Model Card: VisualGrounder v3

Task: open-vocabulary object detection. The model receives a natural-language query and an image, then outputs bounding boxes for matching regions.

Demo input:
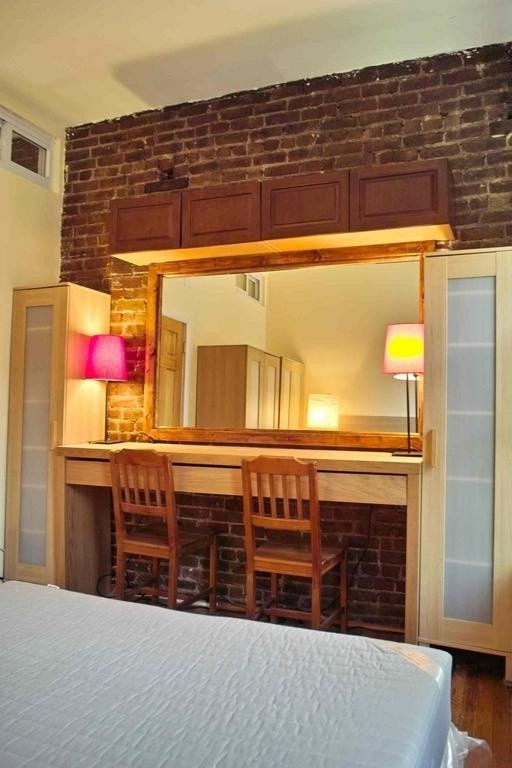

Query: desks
[56,443,422,645]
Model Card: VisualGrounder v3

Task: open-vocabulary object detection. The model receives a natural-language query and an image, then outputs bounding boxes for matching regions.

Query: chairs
[111,448,217,614]
[242,455,347,631]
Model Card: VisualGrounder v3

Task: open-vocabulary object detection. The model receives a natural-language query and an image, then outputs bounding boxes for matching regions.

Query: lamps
[383,324,427,457]
[80,334,123,444]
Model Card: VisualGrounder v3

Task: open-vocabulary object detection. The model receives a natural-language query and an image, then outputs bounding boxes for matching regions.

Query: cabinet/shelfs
[349,157,456,241]
[260,172,350,241]
[108,193,181,256]
[181,181,260,251]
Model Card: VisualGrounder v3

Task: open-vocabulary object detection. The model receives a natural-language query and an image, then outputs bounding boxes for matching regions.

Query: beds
[0,580,457,767]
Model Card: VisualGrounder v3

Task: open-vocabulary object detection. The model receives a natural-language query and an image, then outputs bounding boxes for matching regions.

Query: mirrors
[143,237,438,452]
